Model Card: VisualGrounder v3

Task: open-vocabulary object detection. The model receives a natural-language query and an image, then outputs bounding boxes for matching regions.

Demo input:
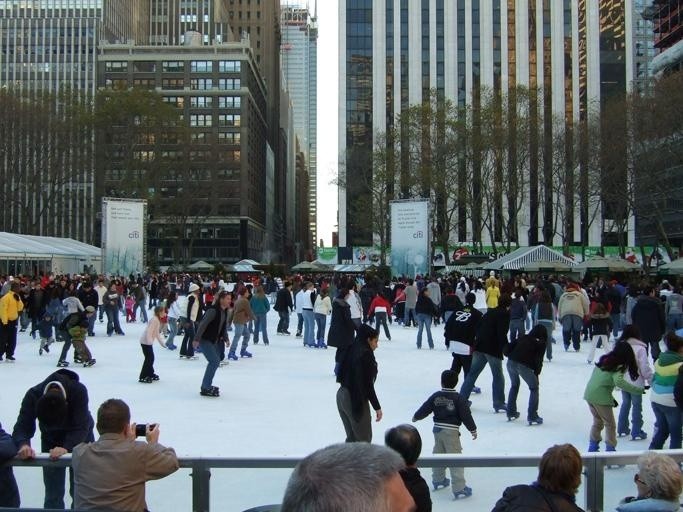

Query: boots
[303,339,325,346]
[228,352,238,359]
[240,349,253,355]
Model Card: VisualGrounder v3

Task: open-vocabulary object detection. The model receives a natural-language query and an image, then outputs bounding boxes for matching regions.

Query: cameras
[135,424,152,436]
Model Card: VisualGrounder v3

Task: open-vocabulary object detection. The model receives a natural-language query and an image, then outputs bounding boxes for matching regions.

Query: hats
[189,282,200,292]
[86,306,95,313]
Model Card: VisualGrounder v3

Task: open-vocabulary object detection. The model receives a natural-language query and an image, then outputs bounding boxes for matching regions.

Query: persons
[0,269,683,512]
[625,247,637,264]
[358,250,366,260]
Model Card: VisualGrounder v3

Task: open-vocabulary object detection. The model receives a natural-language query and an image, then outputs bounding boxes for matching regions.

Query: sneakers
[139,374,159,380]
[632,430,646,437]
[495,404,508,410]
[433,478,449,486]
[507,412,519,418]
[618,428,630,433]
[473,387,480,392]
[529,416,542,421]
[45,344,49,352]
[277,328,282,332]
[296,330,301,336]
[455,487,471,494]
[58,361,68,365]
[6,355,15,360]
[166,344,176,349]
[283,330,290,334]
[202,386,220,395]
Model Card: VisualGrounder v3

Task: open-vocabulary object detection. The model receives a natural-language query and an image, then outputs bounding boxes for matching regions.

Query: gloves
[14,294,20,301]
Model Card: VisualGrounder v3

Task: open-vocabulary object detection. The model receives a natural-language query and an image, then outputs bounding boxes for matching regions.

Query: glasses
[634,473,646,485]
